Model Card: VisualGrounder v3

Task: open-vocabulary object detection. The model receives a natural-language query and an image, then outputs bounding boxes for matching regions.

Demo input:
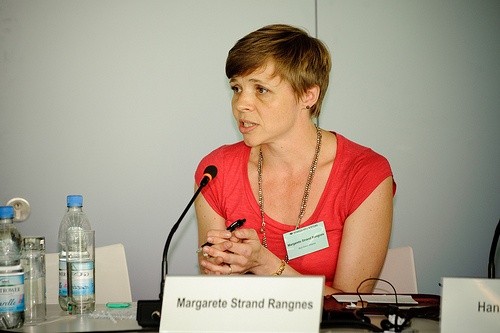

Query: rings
[229,266,232,273]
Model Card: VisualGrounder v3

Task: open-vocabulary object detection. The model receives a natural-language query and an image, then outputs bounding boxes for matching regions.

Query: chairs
[374,243,419,296]
[43,245,132,305]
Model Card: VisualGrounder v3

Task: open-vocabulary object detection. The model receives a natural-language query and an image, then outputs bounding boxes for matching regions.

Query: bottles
[0,205,25,330]
[58,195,92,311]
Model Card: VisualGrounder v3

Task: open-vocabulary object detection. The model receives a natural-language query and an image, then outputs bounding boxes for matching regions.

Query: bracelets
[273,260,286,276]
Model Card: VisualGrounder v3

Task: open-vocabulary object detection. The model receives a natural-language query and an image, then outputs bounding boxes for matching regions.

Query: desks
[0,301,440,333]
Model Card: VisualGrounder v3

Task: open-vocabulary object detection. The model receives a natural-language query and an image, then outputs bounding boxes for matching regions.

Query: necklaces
[257,128,321,263]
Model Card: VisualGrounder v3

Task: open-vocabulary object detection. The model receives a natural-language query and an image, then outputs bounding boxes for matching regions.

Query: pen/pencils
[196,218,246,253]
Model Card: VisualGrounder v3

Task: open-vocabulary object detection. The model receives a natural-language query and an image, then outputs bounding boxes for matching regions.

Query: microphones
[135,165,218,329]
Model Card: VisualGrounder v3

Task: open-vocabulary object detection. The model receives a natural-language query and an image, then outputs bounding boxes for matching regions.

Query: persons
[195,24,397,297]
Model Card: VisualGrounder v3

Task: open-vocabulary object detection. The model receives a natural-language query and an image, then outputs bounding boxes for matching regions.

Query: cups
[21,236,46,326]
[66,231,96,315]
